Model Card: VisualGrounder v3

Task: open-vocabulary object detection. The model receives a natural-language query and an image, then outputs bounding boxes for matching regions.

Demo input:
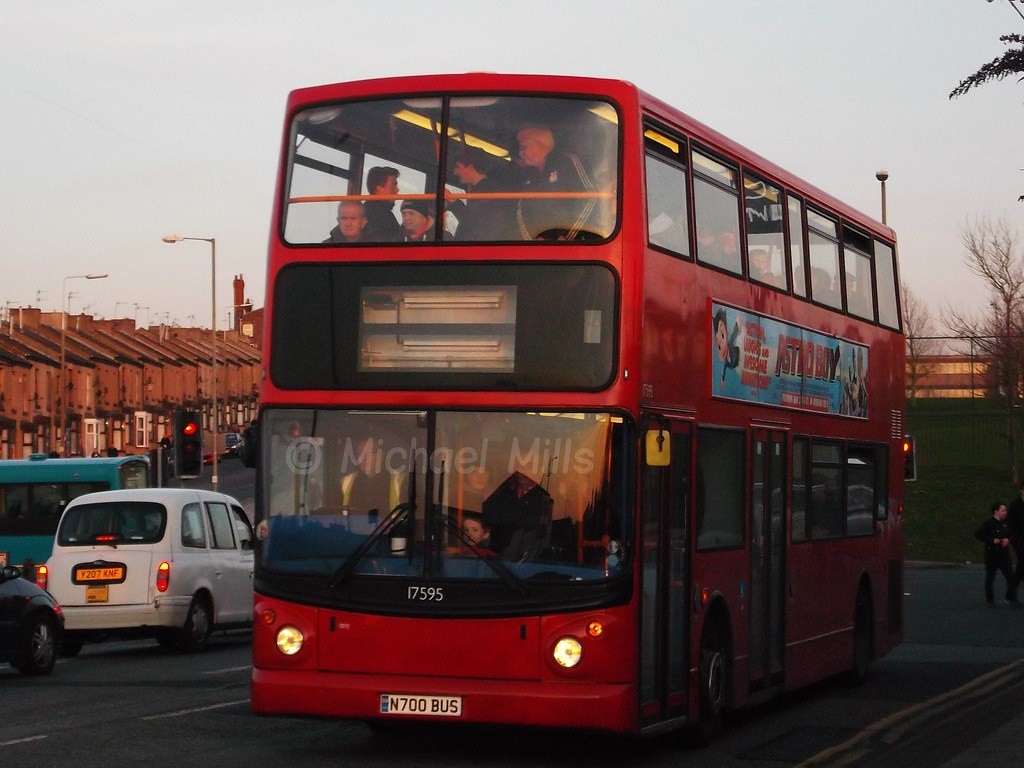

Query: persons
[451,457,496,517]
[444,517,496,557]
[750,249,783,288]
[515,127,601,241]
[482,453,553,561]
[334,436,408,517]
[321,201,382,243]
[363,167,400,242]
[1004,488,1024,602]
[388,199,454,241]
[974,502,1014,607]
[443,147,515,240]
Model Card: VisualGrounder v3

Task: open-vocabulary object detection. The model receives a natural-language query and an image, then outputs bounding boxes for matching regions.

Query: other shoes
[986,600,996,608]
[1004,600,1022,608]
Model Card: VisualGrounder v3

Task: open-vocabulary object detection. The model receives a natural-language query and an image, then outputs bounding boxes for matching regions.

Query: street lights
[163,236,218,491]
[223,304,253,432]
[59,273,108,459]
[875,172,889,226]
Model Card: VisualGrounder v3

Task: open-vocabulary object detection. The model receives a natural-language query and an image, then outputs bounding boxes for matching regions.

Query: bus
[0,453,150,578]
[253,72,907,741]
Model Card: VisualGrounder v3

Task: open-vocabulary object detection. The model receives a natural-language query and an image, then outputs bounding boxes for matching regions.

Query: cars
[202,451,222,465]
[221,433,245,459]
[0,564,65,678]
[36,487,255,654]
[846,485,886,535]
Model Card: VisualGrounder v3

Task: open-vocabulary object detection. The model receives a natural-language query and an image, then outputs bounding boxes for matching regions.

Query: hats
[403,199,428,216]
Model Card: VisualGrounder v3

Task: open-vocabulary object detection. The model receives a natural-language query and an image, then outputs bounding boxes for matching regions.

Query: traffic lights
[175,412,203,478]
[905,435,917,482]
